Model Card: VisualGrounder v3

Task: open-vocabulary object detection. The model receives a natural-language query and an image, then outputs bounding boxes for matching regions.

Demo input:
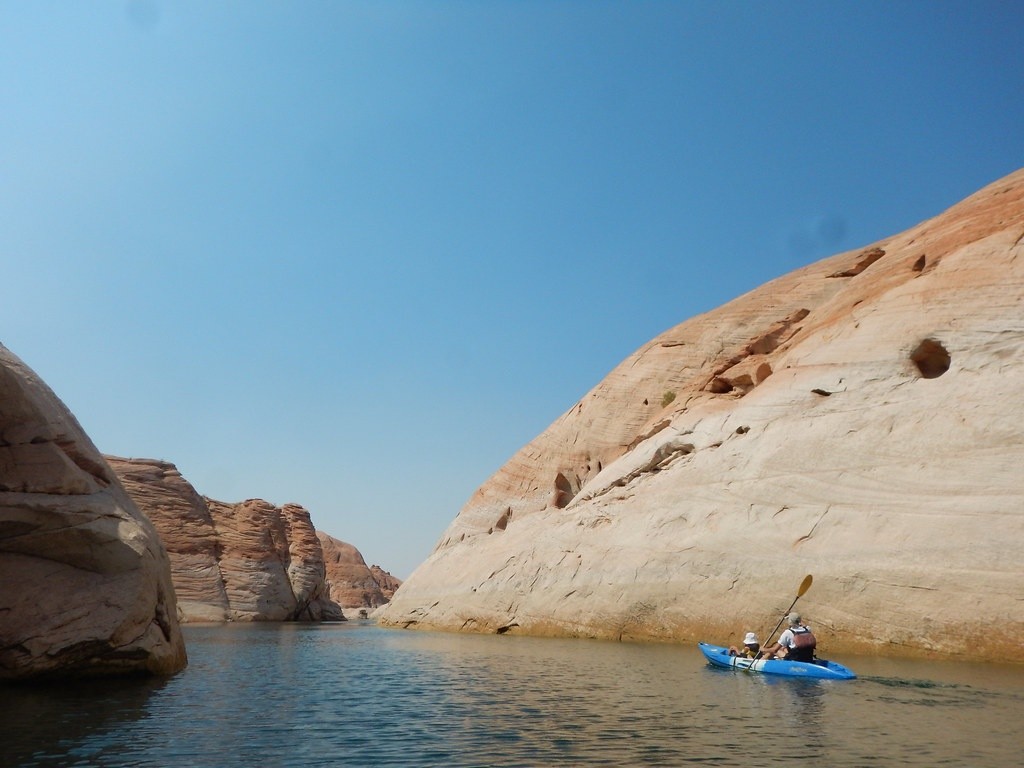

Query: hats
[742,632,758,644]
[785,612,802,624]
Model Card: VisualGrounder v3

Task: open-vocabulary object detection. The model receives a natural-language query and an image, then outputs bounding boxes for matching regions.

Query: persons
[759,612,816,663]
[729,633,763,659]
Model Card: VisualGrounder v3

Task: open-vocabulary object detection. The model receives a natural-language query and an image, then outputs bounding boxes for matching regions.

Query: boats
[696,641,856,679]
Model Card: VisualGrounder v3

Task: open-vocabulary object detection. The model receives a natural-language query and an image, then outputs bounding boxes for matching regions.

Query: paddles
[743,573,813,673]
